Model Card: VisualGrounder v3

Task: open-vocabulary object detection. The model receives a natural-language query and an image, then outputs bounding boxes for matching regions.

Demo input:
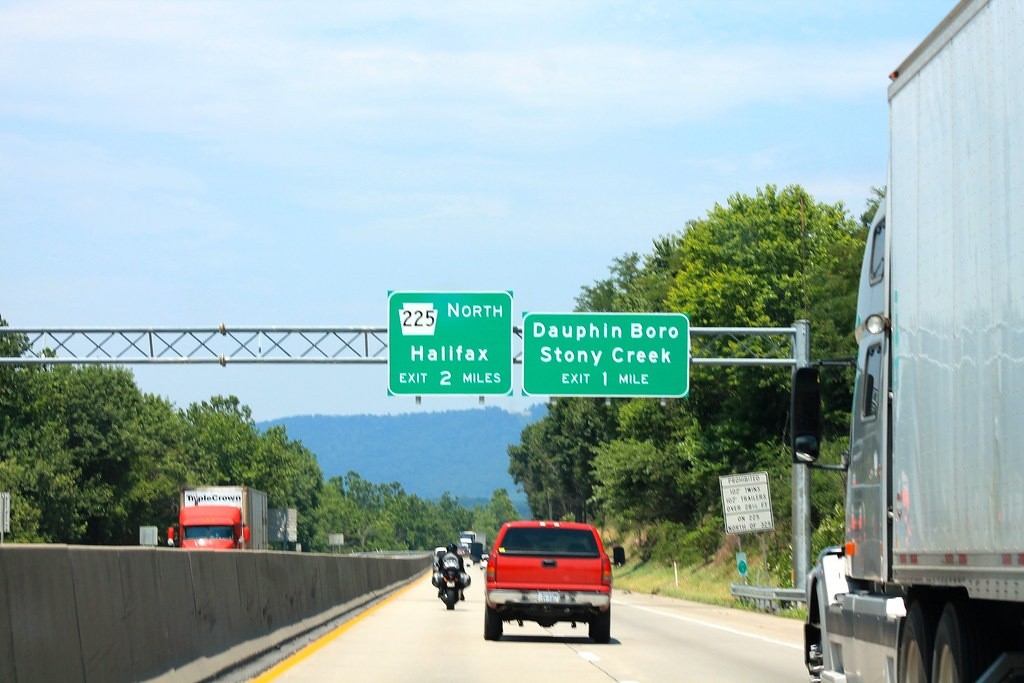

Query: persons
[437,544,466,600]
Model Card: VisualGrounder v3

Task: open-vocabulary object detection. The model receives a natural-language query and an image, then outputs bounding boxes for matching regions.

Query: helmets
[446,543,457,552]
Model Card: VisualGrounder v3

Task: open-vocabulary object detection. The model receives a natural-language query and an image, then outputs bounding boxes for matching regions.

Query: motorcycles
[433,553,461,610]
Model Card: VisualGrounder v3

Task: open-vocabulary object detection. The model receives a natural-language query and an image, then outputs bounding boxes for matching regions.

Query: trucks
[790,0,1022,683]
[167,485,268,551]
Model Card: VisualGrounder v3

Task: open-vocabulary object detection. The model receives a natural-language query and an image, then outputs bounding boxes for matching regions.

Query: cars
[469,519,625,641]
